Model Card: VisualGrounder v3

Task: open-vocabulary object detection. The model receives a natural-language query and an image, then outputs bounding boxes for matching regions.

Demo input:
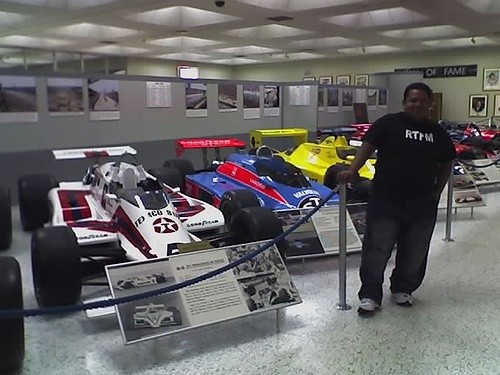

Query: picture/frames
[493,94,500,117]
[354,74,369,86]
[469,94,488,117]
[319,76,333,85]
[483,68,500,91]
[336,75,351,85]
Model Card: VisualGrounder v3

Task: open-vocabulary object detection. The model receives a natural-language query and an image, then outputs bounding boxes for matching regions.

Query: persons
[227,241,296,312]
[487,72,498,86]
[336,82,458,313]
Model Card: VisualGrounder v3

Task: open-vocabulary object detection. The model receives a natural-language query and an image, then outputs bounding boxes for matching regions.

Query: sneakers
[390,291,414,306]
[357,297,383,315]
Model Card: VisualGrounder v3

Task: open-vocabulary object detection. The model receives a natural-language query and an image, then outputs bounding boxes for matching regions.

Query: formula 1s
[249,127,379,199]
[18,145,286,308]
[350,119,500,171]
[175,140,372,263]
[125,302,183,331]
[114,272,167,290]
